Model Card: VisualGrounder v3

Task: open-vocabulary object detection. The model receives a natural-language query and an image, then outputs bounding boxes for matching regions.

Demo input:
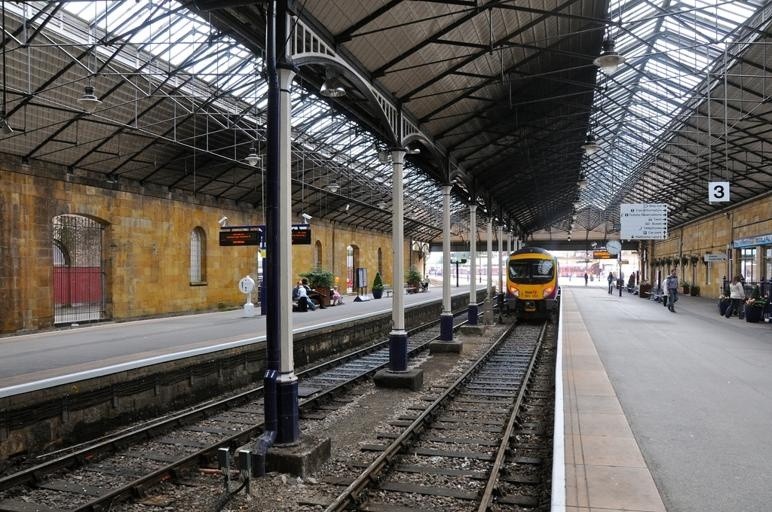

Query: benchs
[385,289,393,298]
[330,295,344,304]
[405,287,416,295]
[615,280,668,308]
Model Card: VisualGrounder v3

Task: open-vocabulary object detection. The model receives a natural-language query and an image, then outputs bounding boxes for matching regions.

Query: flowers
[716,294,730,303]
[741,282,765,309]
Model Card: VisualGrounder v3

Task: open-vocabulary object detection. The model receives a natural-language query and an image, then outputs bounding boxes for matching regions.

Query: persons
[423,274,431,284]
[607,271,613,295]
[584,272,588,286]
[290,278,344,310]
[723,274,745,319]
[666,268,679,311]
[661,272,670,307]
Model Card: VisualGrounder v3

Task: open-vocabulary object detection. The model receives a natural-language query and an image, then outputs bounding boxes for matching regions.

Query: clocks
[606,240,622,256]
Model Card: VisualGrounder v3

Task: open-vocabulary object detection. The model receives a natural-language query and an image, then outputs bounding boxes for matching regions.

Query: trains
[505,246,559,322]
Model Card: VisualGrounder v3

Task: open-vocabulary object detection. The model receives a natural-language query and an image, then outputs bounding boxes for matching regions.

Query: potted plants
[404,264,422,294]
[689,284,700,297]
[677,278,689,294]
[298,265,336,309]
[372,270,384,299]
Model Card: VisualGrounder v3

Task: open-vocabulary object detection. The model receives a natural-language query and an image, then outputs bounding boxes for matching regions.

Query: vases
[719,301,728,316]
[745,304,763,323]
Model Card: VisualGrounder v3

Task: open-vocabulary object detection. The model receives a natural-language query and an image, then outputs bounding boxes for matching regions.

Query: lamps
[327,178,341,194]
[244,145,262,169]
[318,68,347,100]
[579,126,604,160]
[589,30,630,75]
[75,83,105,117]
[564,172,592,243]
[376,197,388,210]
